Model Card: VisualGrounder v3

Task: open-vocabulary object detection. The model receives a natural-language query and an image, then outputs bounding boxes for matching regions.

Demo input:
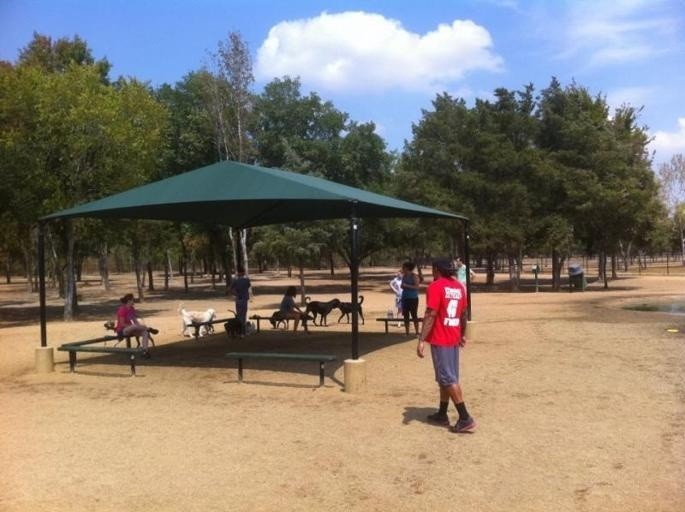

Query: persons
[389,266,407,328]
[454,255,476,289]
[416,254,478,433]
[279,284,309,337]
[225,264,257,339]
[400,261,421,338]
[112,292,158,362]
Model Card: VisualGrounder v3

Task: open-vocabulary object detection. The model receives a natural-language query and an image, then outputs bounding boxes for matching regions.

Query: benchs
[376,318,424,332]
[188,317,235,341]
[58,346,146,376]
[64,334,132,349]
[225,353,335,385]
[249,317,309,332]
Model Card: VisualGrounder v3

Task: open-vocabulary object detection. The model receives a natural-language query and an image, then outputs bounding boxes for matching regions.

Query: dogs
[177,301,216,339]
[104,319,159,350]
[333,296,364,325]
[301,296,341,326]
[269,311,286,329]
[224,309,242,338]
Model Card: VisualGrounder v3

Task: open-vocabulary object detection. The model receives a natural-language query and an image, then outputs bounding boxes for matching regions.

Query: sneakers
[428,414,449,426]
[451,418,474,432]
[149,328,158,334]
